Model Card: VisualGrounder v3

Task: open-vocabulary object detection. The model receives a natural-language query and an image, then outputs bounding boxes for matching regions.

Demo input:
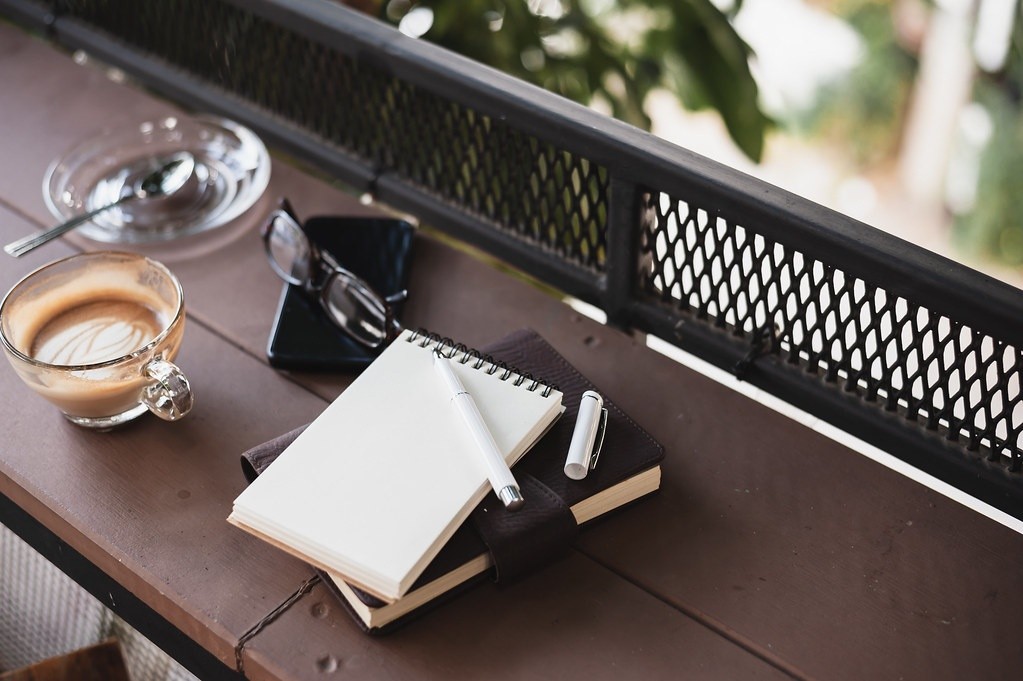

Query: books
[222,322,668,634]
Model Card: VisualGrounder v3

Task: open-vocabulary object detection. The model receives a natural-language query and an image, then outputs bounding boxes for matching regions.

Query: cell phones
[265,213,411,373]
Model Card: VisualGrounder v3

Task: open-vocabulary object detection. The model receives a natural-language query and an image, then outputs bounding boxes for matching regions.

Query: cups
[0,250,194,433]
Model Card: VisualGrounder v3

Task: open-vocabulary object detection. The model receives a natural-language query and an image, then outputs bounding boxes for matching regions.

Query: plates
[42,112,272,247]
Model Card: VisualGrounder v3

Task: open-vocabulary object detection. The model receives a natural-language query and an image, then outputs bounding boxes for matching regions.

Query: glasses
[263,197,408,348]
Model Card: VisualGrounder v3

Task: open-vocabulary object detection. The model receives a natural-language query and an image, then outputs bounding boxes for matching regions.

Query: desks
[0,25,1023,681]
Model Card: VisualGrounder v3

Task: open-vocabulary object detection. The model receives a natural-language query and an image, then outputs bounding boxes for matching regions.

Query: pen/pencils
[430,346,524,510]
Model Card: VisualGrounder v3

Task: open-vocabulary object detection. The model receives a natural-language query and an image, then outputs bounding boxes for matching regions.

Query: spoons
[3,151,195,258]
